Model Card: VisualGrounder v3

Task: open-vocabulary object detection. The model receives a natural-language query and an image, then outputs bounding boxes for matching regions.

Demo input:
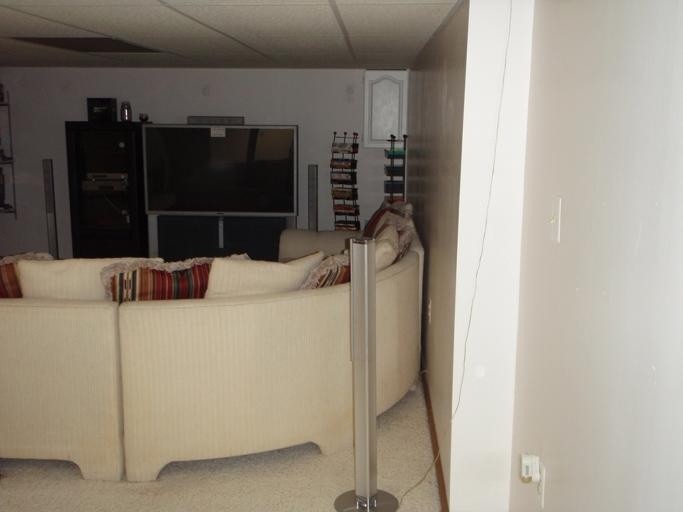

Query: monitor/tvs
[138,122,299,217]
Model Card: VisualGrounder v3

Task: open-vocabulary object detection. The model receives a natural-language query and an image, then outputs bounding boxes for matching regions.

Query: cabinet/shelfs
[62,113,148,257]
[0,90,23,221]
[329,128,361,233]
[383,132,411,202]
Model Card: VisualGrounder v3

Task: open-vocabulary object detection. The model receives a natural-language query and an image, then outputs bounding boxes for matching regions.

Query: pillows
[97,257,207,302]
[360,200,416,268]
[307,252,350,289]
[14,254,162,300]
[0,252,45,300]
[206,252,321,296]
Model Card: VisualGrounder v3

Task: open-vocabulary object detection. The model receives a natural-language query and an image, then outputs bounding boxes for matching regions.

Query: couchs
[0,228,424,483]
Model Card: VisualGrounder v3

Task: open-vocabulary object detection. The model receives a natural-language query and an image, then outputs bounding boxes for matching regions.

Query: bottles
[120,101,132,122]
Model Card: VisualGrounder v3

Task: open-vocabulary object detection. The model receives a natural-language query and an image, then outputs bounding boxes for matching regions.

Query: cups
[138,112,148,121]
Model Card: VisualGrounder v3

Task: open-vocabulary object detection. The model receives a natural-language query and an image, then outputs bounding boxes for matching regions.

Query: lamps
[40,157,58,257]
[333,235,399,512]
[307,163,319,234]
